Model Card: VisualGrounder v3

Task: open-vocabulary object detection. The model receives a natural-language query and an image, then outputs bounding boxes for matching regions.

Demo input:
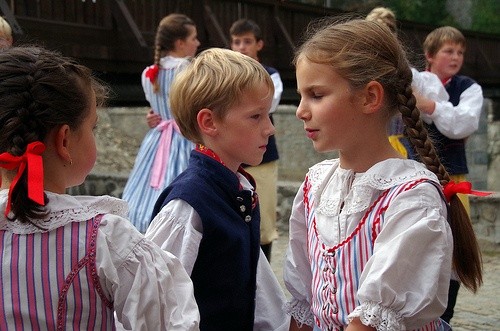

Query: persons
[0,15,13,49]
[280,20,484,331]
[0,45,202,331]
[146,19,283,264]
[366,7,451,126]
[410,27,484,223]
[121,13,200,234]
[144,48,290,331]
